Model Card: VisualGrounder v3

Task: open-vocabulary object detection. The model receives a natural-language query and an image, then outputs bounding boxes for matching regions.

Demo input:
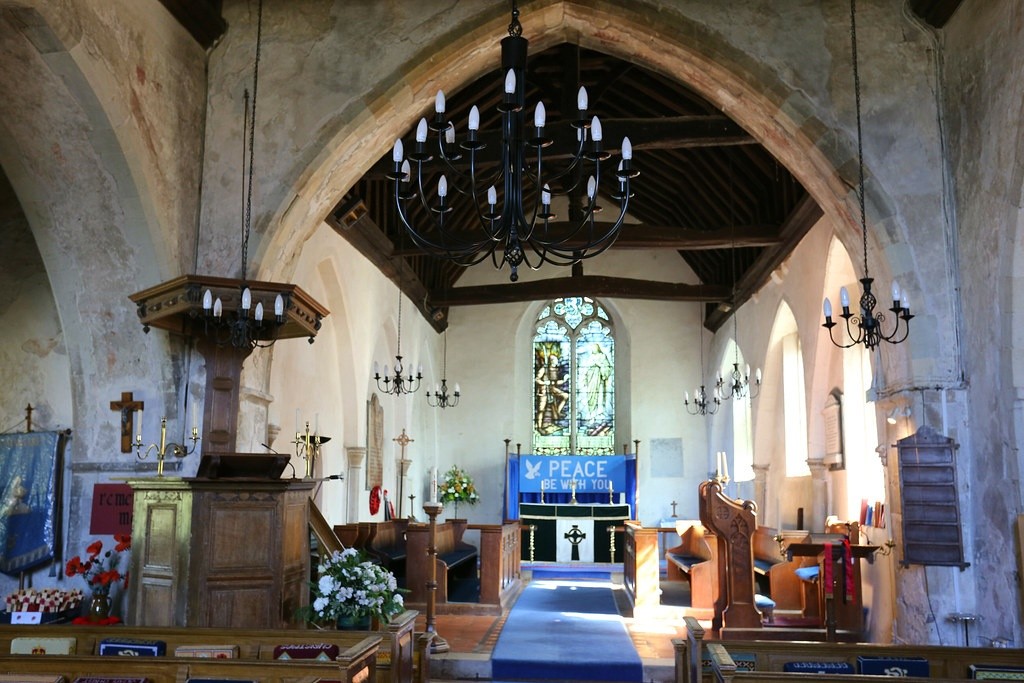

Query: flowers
[66,533,133,594]
[293,544,406,619]
[440,464,484,514]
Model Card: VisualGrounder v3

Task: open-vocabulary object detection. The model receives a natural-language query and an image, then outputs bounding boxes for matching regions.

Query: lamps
[386,0,642,283]
[683,303,721,416]
[199,0,288,350]
[425,330,465,408]
[714,210,762,400]
[821,0,916,350]
[717,302,733,313]
[370,254,426,395]
[431,308,445,322]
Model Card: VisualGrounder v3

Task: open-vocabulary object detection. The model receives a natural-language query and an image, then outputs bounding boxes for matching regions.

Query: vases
[336,607,373,630]
[90,589,119,622]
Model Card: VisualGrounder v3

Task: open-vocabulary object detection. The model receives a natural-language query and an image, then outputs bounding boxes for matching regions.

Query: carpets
[490,567,645,683]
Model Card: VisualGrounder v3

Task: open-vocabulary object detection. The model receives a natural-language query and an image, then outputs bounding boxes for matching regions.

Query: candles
[295,407,302,434]
[716,450,722,477]
[136,406,143,435]
[314,412,320,435]
[541,480,544,489]
[723,451,728,476]
[609,479,613,490]
[193,401,198,426]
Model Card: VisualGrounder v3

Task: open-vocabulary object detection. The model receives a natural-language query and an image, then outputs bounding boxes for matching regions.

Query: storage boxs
[173,644,240,658]
[700,650,759,674]
[98,636,167,657]
[271,642,338,661]
[968,663,1024,680]
[10,636,76,656]
[0,607,82,624]
[782,658,856,674]
[857,655,930,677]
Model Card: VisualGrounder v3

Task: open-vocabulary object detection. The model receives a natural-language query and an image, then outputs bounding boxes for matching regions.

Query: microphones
[262,443,295,477]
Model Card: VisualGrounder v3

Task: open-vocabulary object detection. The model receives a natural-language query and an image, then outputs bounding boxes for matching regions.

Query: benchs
[754,593,777,623]
[667,519,720,608]
[754,525,808,609]
[334,521,406,577]
[409,517,478,604]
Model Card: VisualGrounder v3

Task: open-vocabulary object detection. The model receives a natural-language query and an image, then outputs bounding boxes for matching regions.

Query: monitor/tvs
[197,453,291,479]
